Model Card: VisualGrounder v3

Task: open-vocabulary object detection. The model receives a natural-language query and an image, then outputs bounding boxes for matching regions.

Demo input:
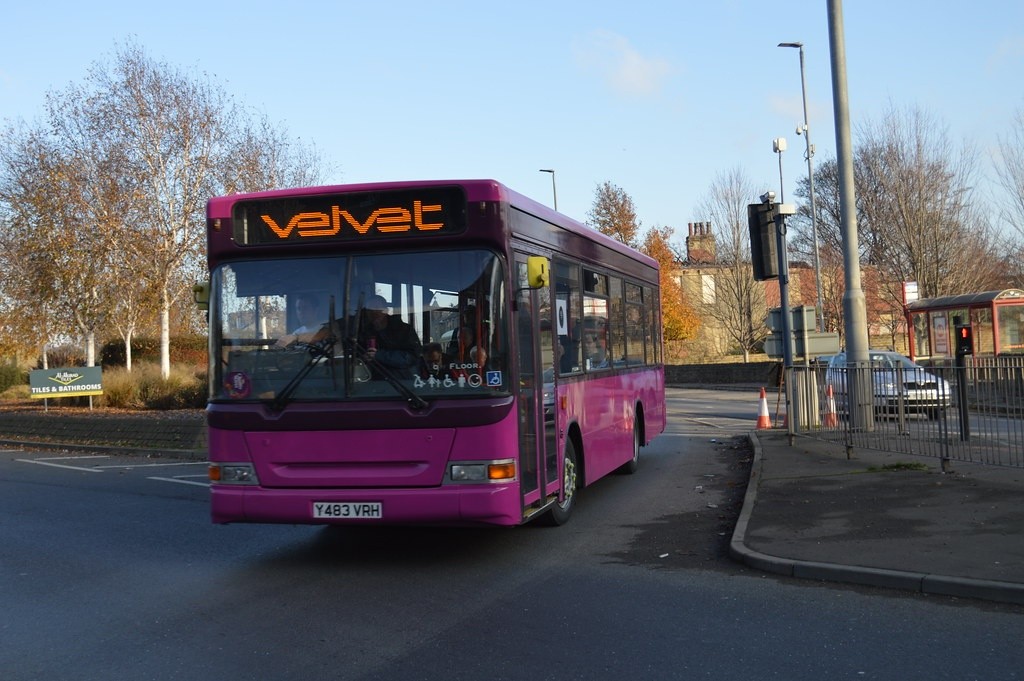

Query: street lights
[539,169,557,211]
[780,43,824,331]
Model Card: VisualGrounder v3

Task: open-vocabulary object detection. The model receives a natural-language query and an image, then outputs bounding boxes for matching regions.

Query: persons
[421,342,469,381]
[627,308,644,339]
[451,328,486,366]
[584,334,608,368]
[276,294,342,357]
[451,306,476,340]
[560,336,578,373]
[366,296,419,380]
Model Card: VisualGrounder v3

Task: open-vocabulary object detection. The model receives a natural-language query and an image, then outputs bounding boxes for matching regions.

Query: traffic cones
[757,387,772,429]
[782,413,789,429]
[823,384,839,426]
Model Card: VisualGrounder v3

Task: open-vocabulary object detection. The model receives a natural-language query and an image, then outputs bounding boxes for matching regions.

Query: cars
[829,351,952,420]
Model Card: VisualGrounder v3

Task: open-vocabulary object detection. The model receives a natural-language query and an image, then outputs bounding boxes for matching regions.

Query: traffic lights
[958,325,974,355]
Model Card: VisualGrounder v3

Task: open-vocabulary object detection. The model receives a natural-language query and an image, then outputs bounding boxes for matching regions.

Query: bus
[192,180,667,528]
